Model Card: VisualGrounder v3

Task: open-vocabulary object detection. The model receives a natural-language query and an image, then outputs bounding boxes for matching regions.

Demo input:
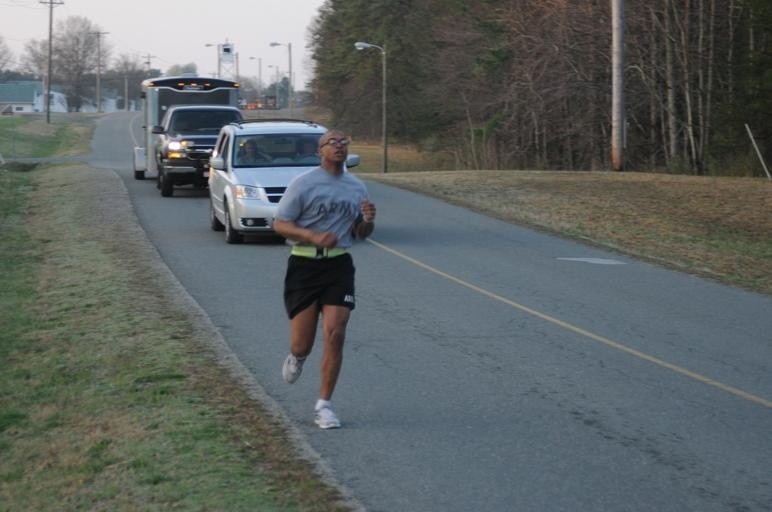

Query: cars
[152,105,245,197]
[208,120,360,244]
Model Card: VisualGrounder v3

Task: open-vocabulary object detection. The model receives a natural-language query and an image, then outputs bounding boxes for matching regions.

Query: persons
[273,129,376,429]
[237,140,270,166]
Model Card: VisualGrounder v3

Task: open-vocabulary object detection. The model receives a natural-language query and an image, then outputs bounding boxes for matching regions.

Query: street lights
[268,64,279,109]
[205,44,221,75]
[270,42,293,120]
[250,56,261,100]
[355,42,389,174]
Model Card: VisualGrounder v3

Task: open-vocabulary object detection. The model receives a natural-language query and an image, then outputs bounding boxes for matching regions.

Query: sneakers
[315,404,340,430]
[282,353,306,384]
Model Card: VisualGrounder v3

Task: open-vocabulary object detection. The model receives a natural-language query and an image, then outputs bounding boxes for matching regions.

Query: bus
[132,77,239,180]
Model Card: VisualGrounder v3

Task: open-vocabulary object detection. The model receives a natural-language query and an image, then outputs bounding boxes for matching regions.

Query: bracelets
[363,216,374,224]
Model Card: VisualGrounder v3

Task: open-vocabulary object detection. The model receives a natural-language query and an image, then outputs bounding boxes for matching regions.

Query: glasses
[320,137,349,148]
